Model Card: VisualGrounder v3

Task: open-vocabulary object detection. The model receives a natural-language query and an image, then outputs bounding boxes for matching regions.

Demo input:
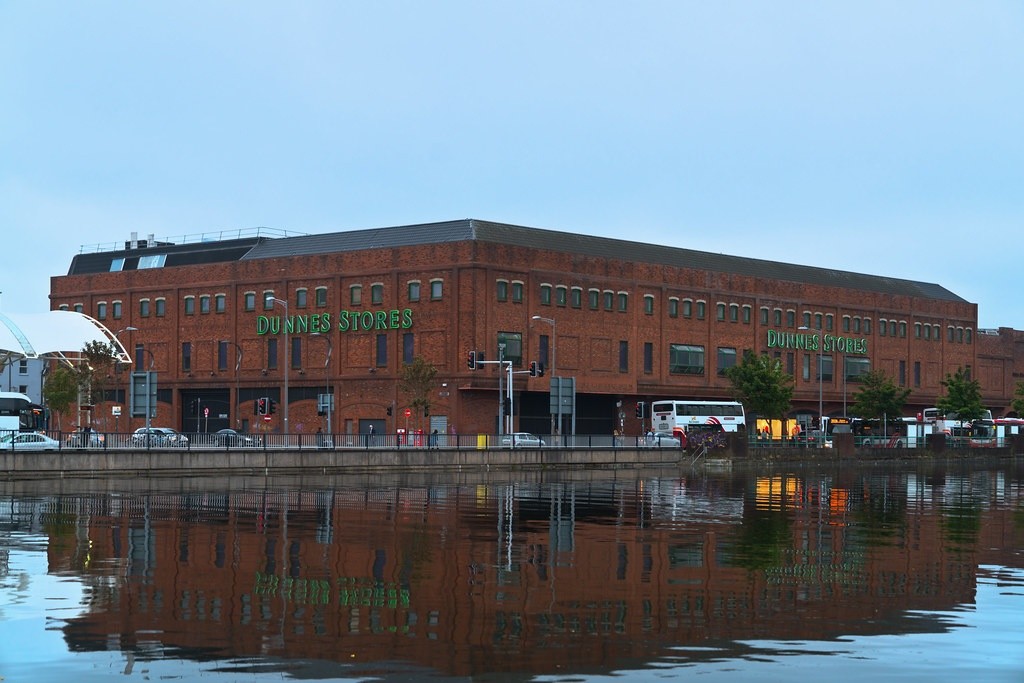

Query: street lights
[532,316,556,447]
[221,341,242,433]
[309,332,332,448]
[265,296,288,447]
[798,326,824,447]
[133,347,154,449]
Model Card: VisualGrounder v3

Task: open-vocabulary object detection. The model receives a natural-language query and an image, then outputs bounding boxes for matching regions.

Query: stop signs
[404,409,410,416]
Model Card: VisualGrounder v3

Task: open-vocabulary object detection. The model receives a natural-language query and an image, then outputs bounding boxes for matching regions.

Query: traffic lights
[503,398,511,415]
[635,402,643,419]
[529,360,536,376]
[259,397,276,416]
[387,405,391,416]
[424,408,428,417]
[468,351,475,369]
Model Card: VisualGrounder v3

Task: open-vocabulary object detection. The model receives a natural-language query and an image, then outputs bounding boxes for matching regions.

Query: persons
[369,425,375,438]
[80,423,94,448]
[296,422,304,432]
[431,430,440,449]
[645,427,656,446]
[316,427,328,447]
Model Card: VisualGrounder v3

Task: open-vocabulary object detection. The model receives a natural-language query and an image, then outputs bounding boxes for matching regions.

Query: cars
[68,429,105,447]
[0,433,59,450]
[210,429,261,448]
[131,427,189,447]
[502,432,545,447]
[797,430,833,448]
[636,432,681,446]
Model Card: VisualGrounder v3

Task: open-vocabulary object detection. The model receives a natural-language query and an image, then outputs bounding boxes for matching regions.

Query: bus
[651,399,746,436]
[817,408,1024,448]
[0,392,46,443]
